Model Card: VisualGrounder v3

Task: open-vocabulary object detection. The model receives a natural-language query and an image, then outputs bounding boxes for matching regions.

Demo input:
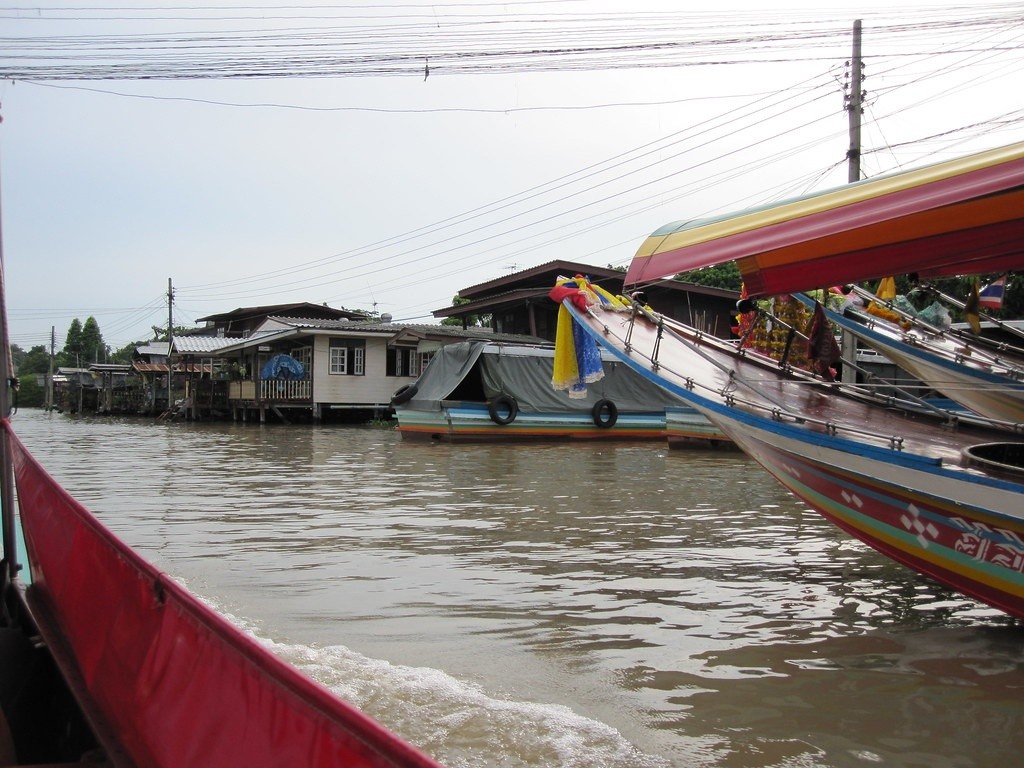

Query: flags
[978,273,1007,308]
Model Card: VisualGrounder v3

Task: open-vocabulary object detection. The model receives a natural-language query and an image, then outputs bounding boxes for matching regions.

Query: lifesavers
[592,399,618,428]
[489,394,518,424]
[391,383,418,405]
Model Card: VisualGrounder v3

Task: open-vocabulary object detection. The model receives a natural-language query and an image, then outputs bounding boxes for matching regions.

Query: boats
[391,336,978,453]
[549,140,1023,626]
